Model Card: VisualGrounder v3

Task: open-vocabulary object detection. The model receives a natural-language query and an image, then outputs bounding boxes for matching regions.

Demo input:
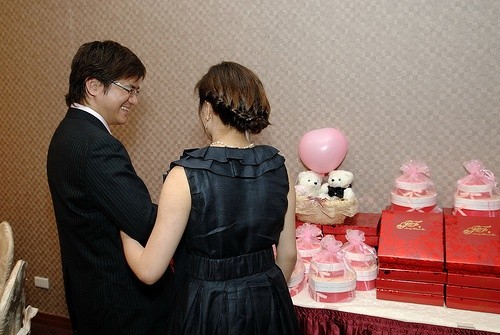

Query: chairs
[0,219,39,335]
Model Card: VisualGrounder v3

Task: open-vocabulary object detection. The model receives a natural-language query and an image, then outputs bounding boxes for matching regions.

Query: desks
[289,281,500,335]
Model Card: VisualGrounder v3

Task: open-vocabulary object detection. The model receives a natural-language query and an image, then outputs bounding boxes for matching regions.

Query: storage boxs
[294,204,500,314]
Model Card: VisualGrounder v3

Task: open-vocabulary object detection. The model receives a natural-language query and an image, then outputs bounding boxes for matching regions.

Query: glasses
[110,79,139,97]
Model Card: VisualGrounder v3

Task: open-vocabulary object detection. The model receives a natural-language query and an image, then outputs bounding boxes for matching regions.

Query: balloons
[298,127,348,174]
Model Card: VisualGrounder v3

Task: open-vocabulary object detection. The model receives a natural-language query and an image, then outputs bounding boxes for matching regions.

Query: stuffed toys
[322,170,355,200]
[295,171,324,198]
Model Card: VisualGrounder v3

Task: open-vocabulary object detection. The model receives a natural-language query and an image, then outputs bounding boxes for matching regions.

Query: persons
[119,62,302,335]
[46,40,174,335]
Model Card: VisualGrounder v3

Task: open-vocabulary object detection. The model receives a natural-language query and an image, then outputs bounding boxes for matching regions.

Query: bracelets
[210,142,254,148]
